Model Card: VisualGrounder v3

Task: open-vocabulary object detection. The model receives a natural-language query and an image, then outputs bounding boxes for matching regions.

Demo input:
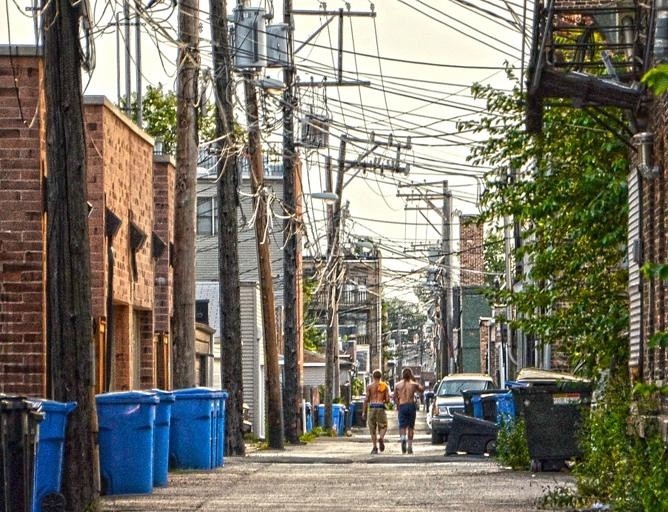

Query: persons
[360,370,391,455]
[393,369,425,454]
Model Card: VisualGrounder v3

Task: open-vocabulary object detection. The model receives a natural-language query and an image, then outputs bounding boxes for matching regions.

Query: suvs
[430,368,497,445]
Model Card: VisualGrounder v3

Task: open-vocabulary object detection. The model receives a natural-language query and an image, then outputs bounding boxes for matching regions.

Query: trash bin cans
[445,381,593,473]
[23,397,78,512]
[95,386,229,495]
[303,402,367,434]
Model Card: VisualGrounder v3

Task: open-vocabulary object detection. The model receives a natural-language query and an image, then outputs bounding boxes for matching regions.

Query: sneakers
[401,440,413,454]
[371,439,384,454]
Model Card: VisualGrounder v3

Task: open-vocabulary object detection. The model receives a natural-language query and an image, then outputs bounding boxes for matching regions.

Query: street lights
[299,192,338,200]
